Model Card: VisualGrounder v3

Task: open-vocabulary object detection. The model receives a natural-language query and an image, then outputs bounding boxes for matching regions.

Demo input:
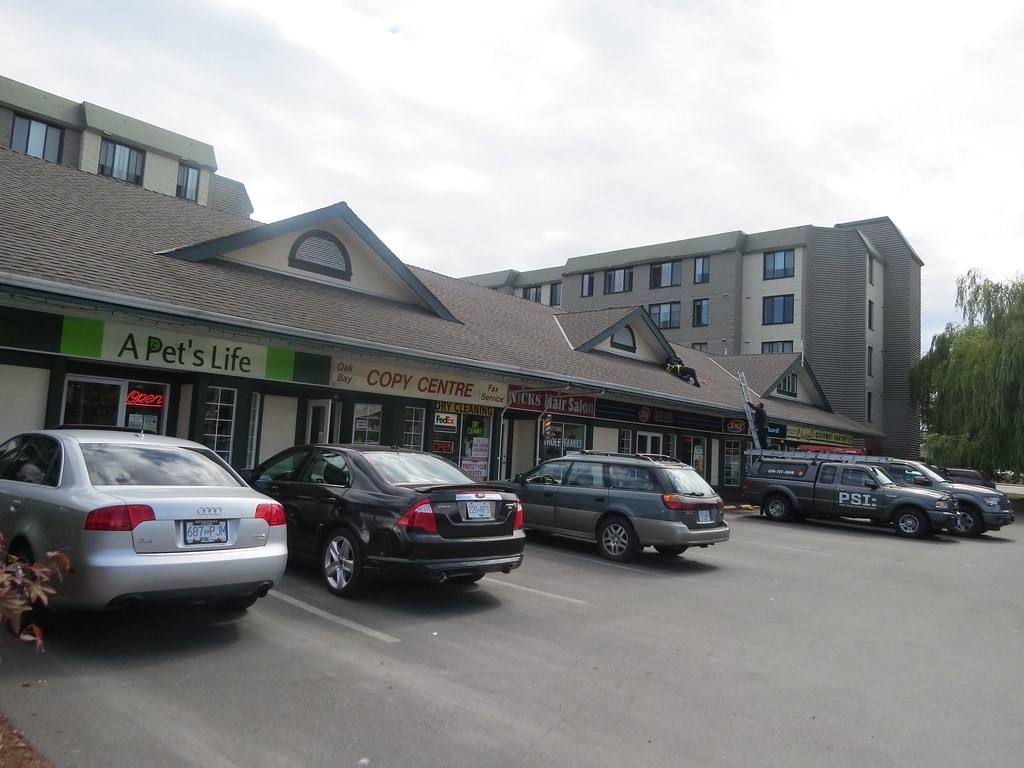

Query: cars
[0,423,288,627]
[243,443,527,598]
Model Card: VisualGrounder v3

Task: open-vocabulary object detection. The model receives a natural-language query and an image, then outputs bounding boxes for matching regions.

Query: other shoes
[681,376,688,382]
[694,382,701,387]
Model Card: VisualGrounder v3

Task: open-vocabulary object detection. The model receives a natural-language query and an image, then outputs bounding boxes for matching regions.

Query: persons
[665,355,701,387]
[747,400,768,448]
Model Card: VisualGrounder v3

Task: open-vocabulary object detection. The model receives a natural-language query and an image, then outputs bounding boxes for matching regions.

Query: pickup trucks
[743,457,959,539]
[857,457,1015,538]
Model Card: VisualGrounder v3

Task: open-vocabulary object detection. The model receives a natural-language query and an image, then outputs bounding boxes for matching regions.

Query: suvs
[484,450,730,562]
[941,467,995,487]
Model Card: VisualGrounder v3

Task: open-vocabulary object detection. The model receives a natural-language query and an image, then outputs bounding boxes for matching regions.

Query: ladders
[737,371,761,449]
